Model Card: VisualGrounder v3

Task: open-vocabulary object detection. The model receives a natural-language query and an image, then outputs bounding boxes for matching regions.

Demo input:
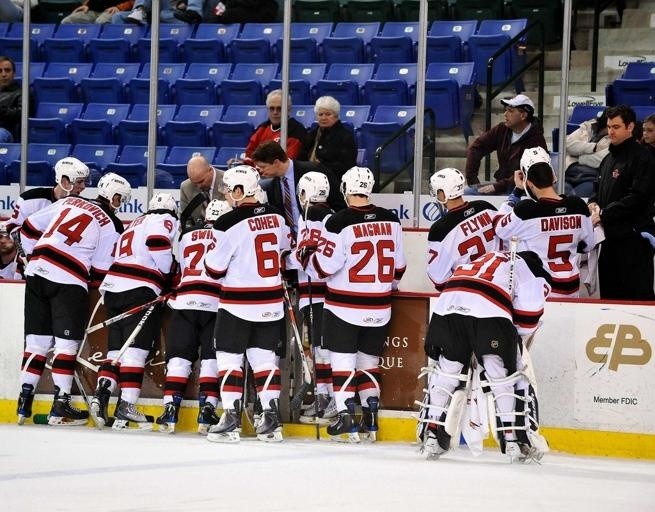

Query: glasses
[270,106,281,113]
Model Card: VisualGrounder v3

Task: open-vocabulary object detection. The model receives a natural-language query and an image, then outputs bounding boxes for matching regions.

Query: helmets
[97,172,132,209]
[340,166,376,198]
[296,171,331,209]
[429,168,465,204]
[0,215,18,239]
[55,157,90,193]
[520,146,557,184]
[205,165,268,222]
[148,192,179,214]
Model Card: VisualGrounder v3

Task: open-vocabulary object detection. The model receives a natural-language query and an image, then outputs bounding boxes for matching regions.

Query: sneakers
[424,436,445,455]
[506,439,531,459]
[127,10,148,24]
[173,9,203,23]
[17,391,34,418]
[298,394,378,435]
[197,402,283,433]
[50,387,182,424]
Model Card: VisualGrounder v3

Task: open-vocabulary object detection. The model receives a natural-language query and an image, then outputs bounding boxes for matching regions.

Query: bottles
[32,413,49,424]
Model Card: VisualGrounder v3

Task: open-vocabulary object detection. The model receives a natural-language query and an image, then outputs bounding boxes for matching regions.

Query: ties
[282,177,294,237]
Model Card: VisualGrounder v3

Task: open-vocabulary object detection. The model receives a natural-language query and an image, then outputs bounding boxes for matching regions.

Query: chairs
[552,60,655,154]
[1,0,549,201]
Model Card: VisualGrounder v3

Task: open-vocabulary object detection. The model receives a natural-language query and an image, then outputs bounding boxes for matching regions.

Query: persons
[0,143,338,444]
[466,93,551,197]
[561,110,614,197]
[284,168,406,443]
[643,113,655,147]
[228,89,307,190]
[1,1,238,24]
[0,55,37,144]
[589,103,654,300]
[294,96,358,213]
[414,146,596,458]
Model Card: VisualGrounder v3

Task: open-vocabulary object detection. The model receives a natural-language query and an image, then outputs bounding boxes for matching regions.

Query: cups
[230,162,238,168]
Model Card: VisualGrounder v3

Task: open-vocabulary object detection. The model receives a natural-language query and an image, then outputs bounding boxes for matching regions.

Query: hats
[501,94,534,116]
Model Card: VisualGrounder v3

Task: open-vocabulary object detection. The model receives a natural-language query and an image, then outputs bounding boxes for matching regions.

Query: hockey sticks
[282,280,312,409]
[12,234,106,430]
[243,359,256,431]
